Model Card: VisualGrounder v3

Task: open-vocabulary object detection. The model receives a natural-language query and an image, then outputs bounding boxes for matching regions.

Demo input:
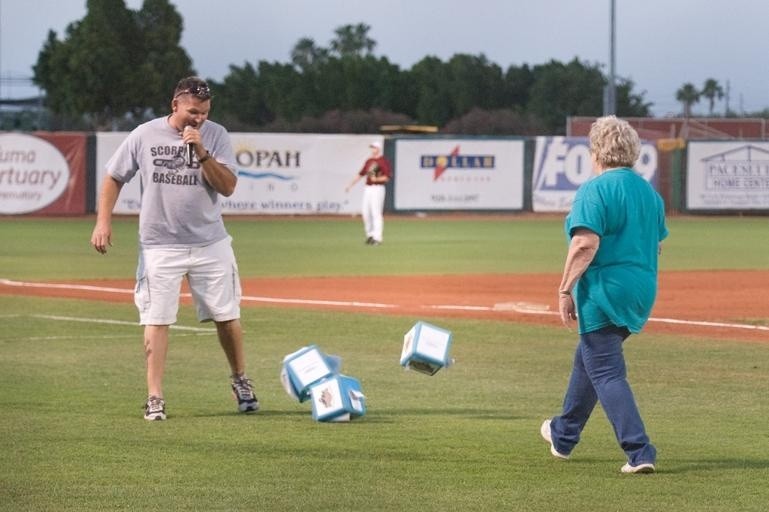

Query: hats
[370,143,379,147]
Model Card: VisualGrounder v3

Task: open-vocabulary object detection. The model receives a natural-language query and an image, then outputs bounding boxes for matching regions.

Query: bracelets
[196,150,212,163]
[559,290,571,295]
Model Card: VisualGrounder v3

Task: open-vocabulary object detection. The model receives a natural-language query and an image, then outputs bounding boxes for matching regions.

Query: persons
[346,142,393,247]
[540,114,671,473]
[89,77,259,423]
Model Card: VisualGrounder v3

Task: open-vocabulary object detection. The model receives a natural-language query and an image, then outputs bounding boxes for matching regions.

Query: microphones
[183,125,195,166]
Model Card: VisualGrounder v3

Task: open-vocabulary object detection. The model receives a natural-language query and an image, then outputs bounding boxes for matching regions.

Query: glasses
[174,87,209,98]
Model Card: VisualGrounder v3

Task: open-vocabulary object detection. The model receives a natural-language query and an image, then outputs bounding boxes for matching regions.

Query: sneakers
[231,377,259,413]
[367,237,379,245]
[145,397,167,421]
[542,420,571,460]
[622,462,654,474]
